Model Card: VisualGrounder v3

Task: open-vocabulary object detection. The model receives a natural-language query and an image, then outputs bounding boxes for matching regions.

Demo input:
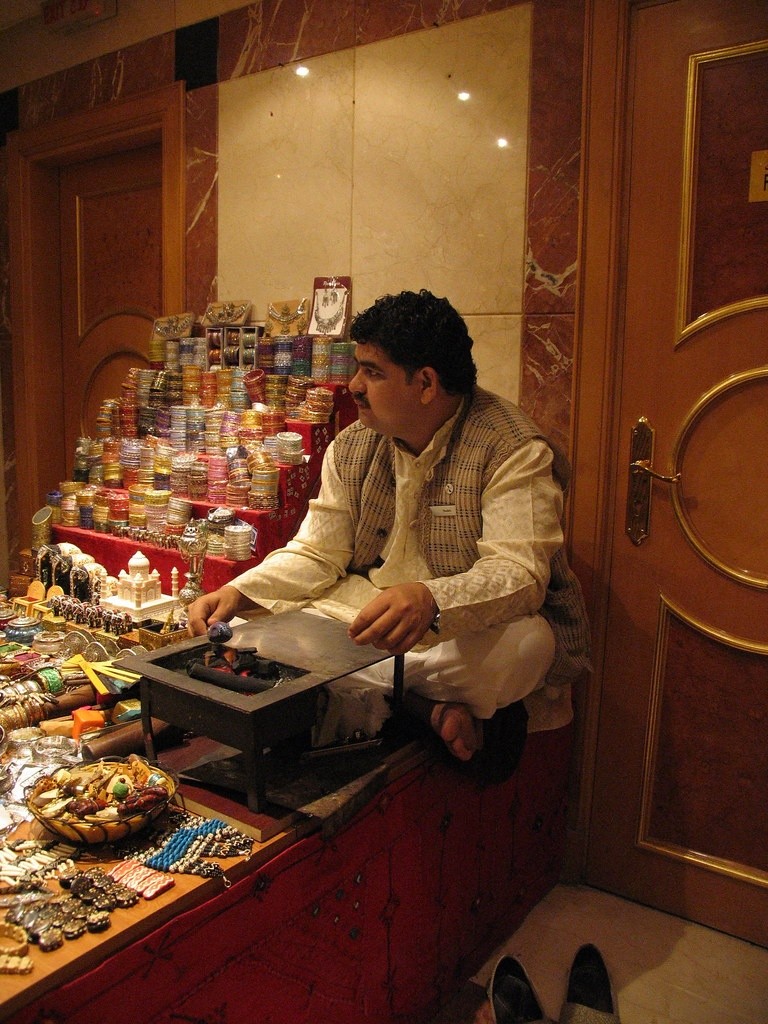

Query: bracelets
[435,610,441,632]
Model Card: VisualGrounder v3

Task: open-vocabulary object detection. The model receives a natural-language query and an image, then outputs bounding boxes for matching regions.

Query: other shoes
[562,940,619,1024]
[488,951,544,1024]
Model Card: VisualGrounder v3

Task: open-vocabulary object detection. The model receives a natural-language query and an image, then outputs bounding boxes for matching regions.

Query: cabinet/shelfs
[51,334,359,597]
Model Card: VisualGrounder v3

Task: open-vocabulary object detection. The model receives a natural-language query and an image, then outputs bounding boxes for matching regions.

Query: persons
[188,288,590,759]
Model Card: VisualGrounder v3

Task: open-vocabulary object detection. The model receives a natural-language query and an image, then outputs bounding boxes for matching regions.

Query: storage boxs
[8,548,36,598]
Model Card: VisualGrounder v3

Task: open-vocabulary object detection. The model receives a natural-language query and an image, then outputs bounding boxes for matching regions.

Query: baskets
[23,756,181,847]
